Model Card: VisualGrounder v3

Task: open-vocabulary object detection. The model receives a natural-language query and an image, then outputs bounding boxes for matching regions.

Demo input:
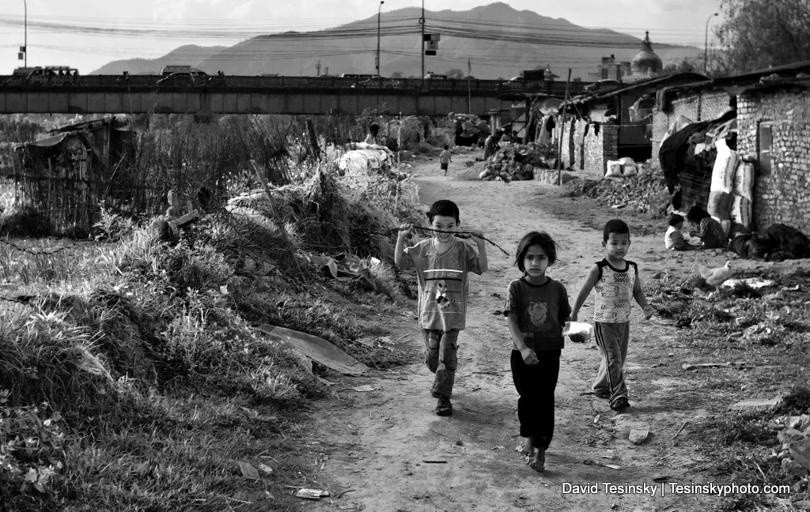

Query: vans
[26,65,78,88]
[160,64,207,75]
[341,75,382,80]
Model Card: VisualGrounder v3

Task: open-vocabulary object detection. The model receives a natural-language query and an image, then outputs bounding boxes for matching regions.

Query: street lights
[376,0,384,73]
[704,12,719,74]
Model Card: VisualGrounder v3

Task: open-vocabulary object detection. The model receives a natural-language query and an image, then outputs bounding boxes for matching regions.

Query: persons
[687,205,726,248]
[365,124,381,145]
[664,211,701,250]
[418,114,431,141]
[447,111,524,160]
[707,123,742,238]
[392,199,489,418]
[503,229,572,472]
[569,219,654,411]
[438,145,453,178]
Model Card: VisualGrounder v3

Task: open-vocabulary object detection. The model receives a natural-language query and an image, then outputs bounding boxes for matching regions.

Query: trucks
[510,69,544,88]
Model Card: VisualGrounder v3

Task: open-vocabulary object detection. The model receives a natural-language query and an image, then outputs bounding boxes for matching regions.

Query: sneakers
[436,398,452,416]
[595,391,630,411]
